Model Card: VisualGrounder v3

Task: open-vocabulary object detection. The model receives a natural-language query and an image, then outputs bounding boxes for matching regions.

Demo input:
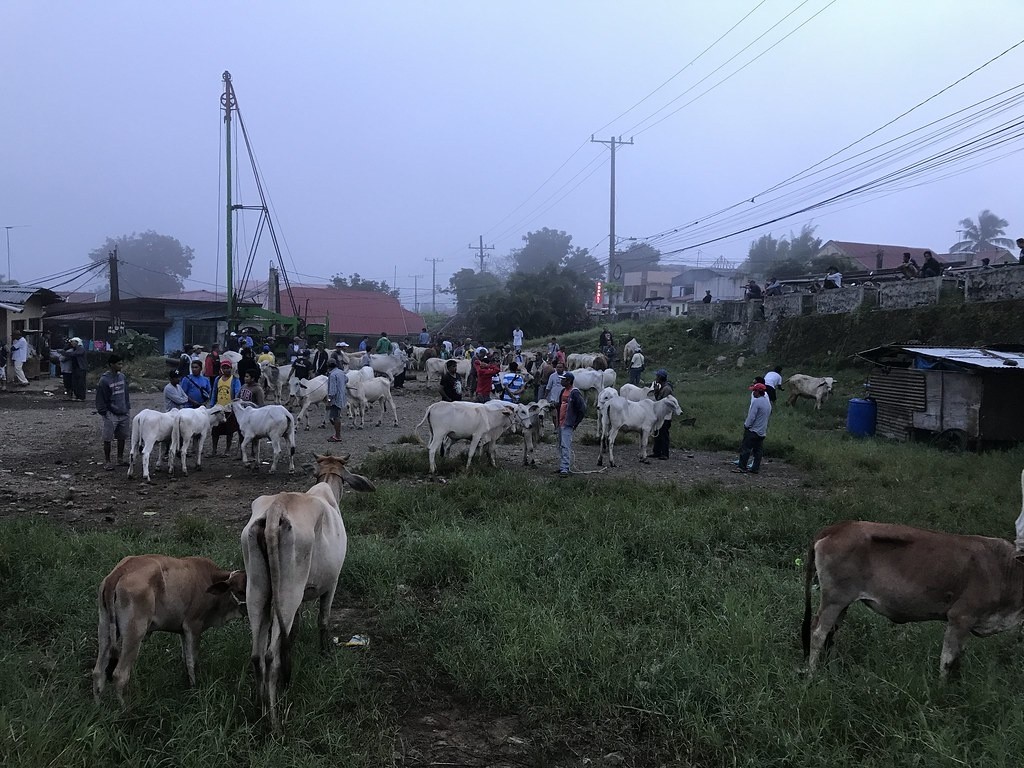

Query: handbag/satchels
[200,388,209,401]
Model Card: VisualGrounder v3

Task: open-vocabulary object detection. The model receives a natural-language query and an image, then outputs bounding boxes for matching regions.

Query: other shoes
[327,434,342,442]
[748,468,758,474]
[731,467,747,472]
[103,461,114,470]
[560,469,569,476]
[650,453,668,460]
[116,459,131,465]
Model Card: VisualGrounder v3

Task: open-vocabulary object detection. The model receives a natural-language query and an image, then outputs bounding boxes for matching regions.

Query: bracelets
[328,399,331,401]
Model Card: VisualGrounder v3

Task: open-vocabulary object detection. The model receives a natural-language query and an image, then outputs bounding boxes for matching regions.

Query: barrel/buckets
[846,397,877,439]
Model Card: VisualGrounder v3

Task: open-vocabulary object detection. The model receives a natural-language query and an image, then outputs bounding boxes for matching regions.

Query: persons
[439,336,525,403]
[36,330,87,402]
[95,356,131,470]
[513,326,524,351]
[648,370,674,460]
[982,258,990,266]
[163,330,276,460]
[598,326,615,369]
[703,290,712,303]
[286,333,349,441]
[823,265,842,288]
[360,332,410,388]
[740,278,821,299]
[1016,238,1024,265]
[732,364,784,474]
[902,251,940,278]
[630,348,645,384]
[550,373,587,474]
[0,330,29,391]
[419,328,430,344]
[531,337,566,434]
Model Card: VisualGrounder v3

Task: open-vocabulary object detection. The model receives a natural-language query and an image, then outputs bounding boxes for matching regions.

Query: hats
[294,337,302,341]
[169,370,181,378]
[654,369,667,377]
[635,348,641,351]
[193,345,204,350]
[184,344,195,350]
[221,359,232,367]
[305,341,350,365]
[267,337,275,341]
[242,329,247,333]
[533,352,542,356]
[558,372,574,381]
[230,332,237,337]
[749,383,766,392]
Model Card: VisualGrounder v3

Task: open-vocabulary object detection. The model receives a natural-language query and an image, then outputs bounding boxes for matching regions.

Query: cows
[566,352,610,371]
[623,337,642,371]
[800,520,1024,685]
[285,366,399,434]
[257,359,292,403]
[423,358,472,390]
[126,398,297,484]
[240,450,377,747]
[785,373,838,411]
[89,553,249,715]
[413,397,556,477]
[596,380,685,469]
[312,348,409,387]
[554,366,617,407]
[391,342,440,370]
